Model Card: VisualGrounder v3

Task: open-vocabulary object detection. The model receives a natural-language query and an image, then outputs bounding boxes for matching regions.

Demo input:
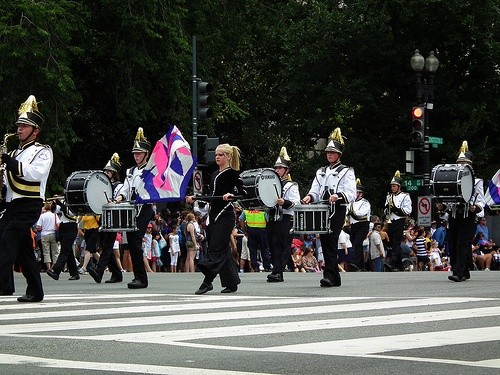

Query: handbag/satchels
[55,230,60,242]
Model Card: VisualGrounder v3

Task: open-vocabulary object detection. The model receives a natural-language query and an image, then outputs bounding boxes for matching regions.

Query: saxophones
[0,132,21,219]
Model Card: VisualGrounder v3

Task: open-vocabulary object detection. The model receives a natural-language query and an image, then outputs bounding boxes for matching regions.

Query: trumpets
[385,192,393,223]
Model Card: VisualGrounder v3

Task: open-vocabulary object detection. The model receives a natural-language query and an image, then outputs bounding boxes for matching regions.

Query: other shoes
[87,266,101,283]
[105,275,123,283]
[253,268,260,272]
[69,275,81,280]
[46,270,60,281]
[267,274,283,283]
[127,280,148,288]
[17,294,44,302]
[319,278,339,287]
[220,285,238,293]
[448,274,471,282]
[265,268,271,272]
[196,281,213,295]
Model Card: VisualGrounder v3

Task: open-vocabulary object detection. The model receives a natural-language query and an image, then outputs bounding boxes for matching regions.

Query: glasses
[311,251,314,253]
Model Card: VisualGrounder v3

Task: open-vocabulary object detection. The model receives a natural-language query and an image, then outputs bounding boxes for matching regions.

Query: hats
[147,223,153,228]
[273,146,290,170]
[131,127,151,153]
[15,94,45,131]
[323,128,344,155]
[355,179,363,193]
[456,141,473,163]
[389,170,402,187]
[102,153,121,174]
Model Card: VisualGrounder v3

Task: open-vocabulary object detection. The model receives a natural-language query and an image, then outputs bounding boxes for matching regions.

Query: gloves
[1,153,19,174]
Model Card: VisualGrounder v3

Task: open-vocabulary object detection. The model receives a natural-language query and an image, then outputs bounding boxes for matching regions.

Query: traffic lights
[205,137,219,165]
[411,106,425,142]
[197,80,213,121]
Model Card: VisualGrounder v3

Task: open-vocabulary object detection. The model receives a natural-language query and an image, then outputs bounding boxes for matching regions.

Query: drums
[64,170,113,216]
[100,204,138,231]
[291,205,331,235]
[237,168,283,211]
[429,164,476,203]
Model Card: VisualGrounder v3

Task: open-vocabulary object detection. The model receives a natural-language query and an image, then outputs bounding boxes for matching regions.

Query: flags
[132,124,197,204]
[483,170,500,208]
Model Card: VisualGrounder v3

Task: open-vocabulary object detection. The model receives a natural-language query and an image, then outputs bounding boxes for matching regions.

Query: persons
[264,147,301,282]
[437,141,485,282]
[302,127,357,288]
[0,95,54,302]
[185,143,248,295]
[0,95,500,273]
[107,127,166,289]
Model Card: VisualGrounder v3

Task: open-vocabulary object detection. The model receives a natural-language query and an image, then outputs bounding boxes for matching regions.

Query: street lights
[412,47,438,195]
